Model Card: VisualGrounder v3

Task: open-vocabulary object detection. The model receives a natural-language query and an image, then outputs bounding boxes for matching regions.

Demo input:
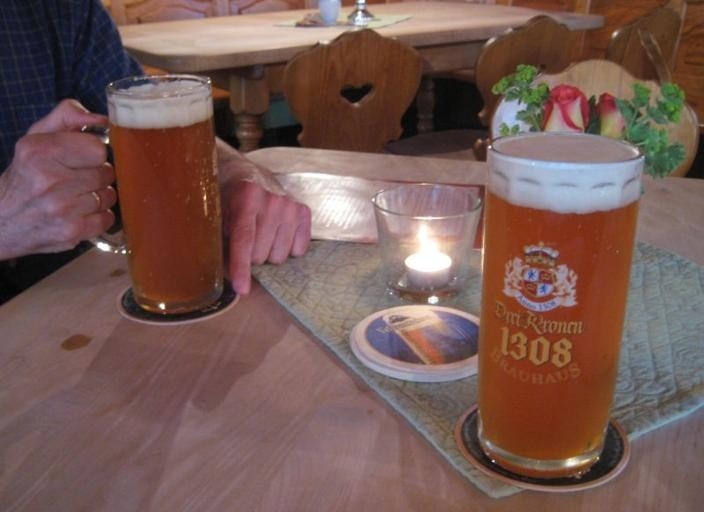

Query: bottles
[319,0,340,24]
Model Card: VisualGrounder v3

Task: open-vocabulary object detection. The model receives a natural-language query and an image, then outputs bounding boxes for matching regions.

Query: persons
[0,0,313,297]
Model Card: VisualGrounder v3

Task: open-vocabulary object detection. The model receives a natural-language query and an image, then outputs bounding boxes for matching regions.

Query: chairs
[606,8,682,83]
[489,58,699,177]
[282,29,424,152]
[474,15,573,127]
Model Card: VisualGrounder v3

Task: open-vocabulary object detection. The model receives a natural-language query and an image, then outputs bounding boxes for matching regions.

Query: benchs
[107,0,328,109]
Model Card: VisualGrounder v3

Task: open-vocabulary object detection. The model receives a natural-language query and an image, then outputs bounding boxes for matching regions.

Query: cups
[372,184,483,301]
[477,131,644,479]
[82,73,225,315]
